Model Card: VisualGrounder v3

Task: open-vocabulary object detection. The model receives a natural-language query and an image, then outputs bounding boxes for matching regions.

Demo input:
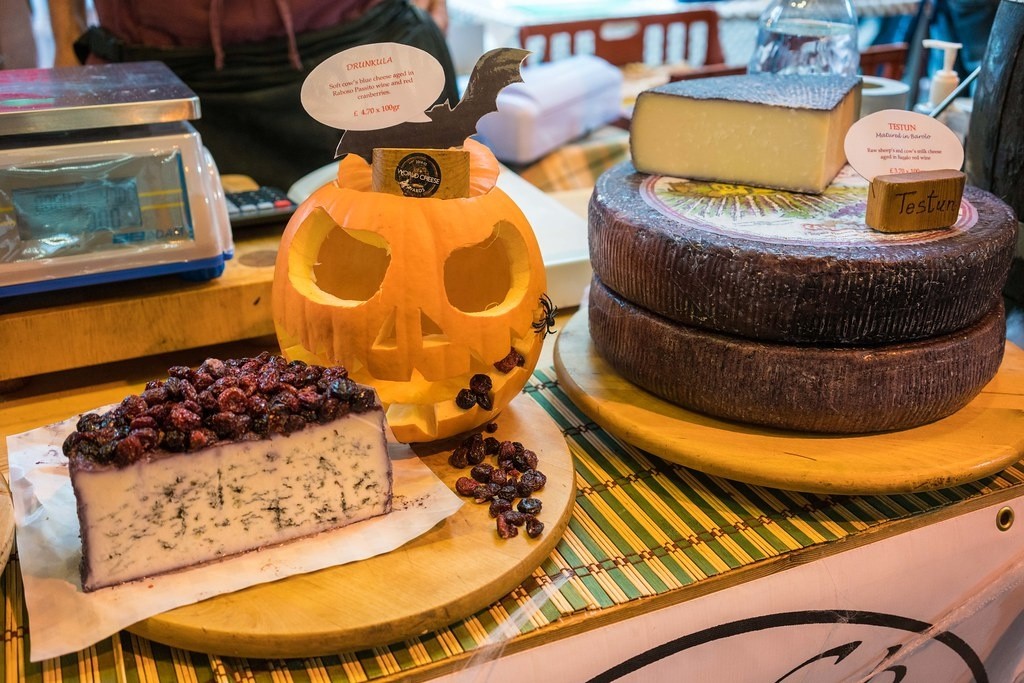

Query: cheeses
[589,270,1008,433]
[589,159,1018,349]
[629,73,863,198]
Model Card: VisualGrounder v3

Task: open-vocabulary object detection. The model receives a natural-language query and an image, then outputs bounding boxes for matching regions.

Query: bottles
[914,36,972,139]
[746,1,860,75]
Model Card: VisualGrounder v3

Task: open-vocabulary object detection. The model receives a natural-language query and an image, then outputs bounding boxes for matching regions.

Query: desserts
[63,347,392,593]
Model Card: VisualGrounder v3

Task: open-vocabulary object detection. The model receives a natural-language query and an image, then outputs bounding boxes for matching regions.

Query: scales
[0,60,235,309]
